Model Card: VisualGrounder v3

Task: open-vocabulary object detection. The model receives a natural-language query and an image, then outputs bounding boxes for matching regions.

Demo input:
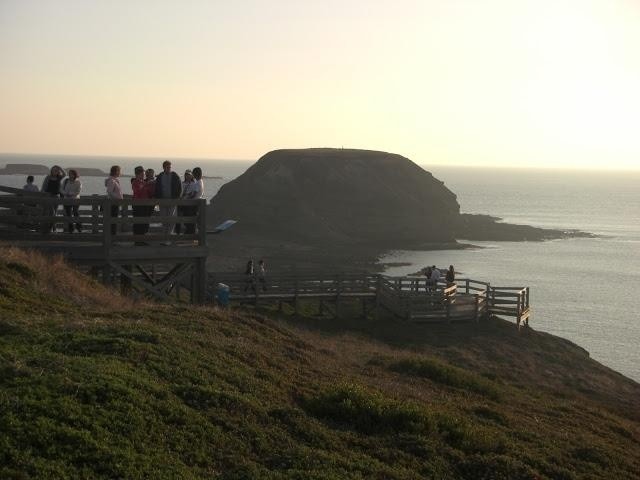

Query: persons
[244,260,256,293]
[424,266,432,292]
[257,260,268,294]
[430,265,440,291]
[445,265,455,288]
[21,159,205,246]
